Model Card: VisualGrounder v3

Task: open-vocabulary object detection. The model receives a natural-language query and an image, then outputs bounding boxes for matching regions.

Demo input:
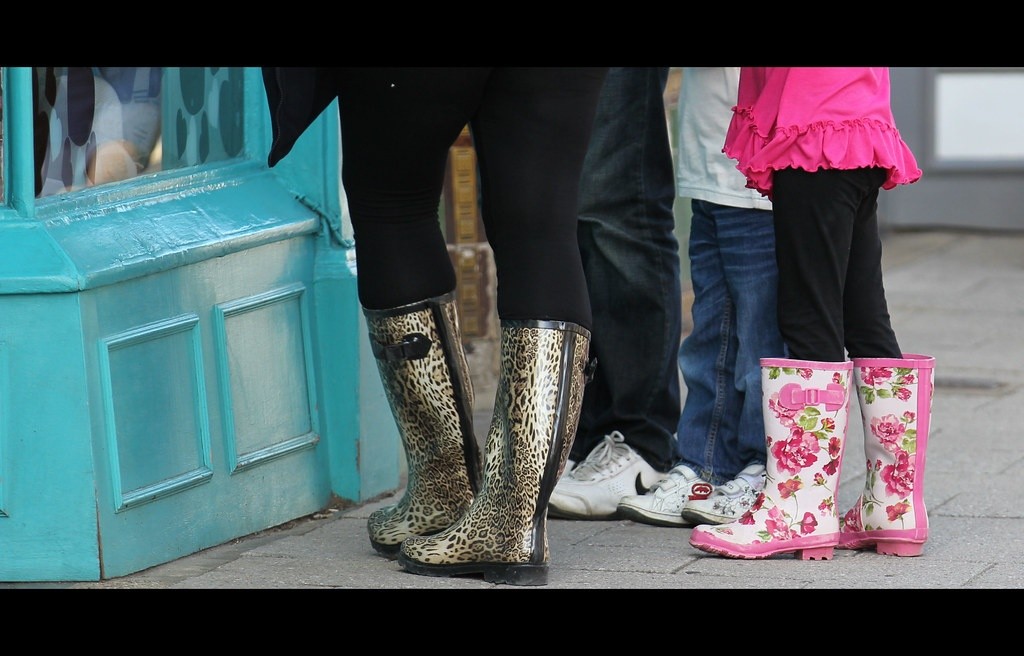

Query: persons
[690,67,935,560]
[548,65,681,520]
[261,65,606,588]
[615,67,787,526]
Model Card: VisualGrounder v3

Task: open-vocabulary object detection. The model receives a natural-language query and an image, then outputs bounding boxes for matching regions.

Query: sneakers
[681,463,769,528]
[616,467,722,528]
[548,443,671,519]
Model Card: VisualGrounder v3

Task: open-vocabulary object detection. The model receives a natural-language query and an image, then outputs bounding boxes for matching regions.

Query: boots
[835,354,935,556]
[688,357,856,560]
[397,317,593,585]
[368,290,486,560]
